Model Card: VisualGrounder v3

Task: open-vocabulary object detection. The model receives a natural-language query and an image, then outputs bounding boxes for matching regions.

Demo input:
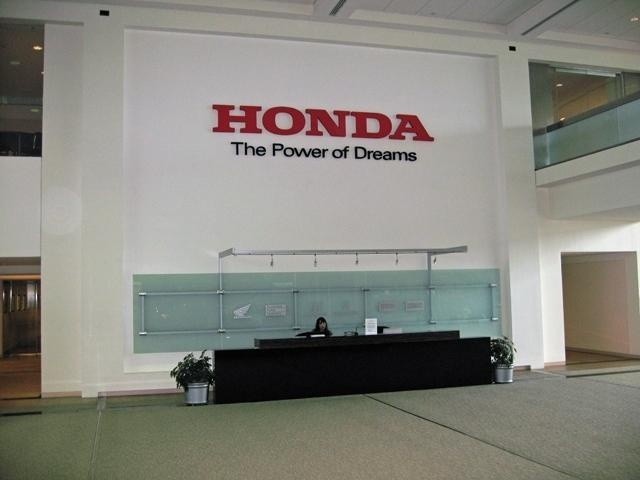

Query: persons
[312,316,332,338]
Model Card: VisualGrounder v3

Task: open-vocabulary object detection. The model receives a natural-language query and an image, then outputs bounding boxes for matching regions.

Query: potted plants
[171,349,213,406]
[490,335,517,384]
